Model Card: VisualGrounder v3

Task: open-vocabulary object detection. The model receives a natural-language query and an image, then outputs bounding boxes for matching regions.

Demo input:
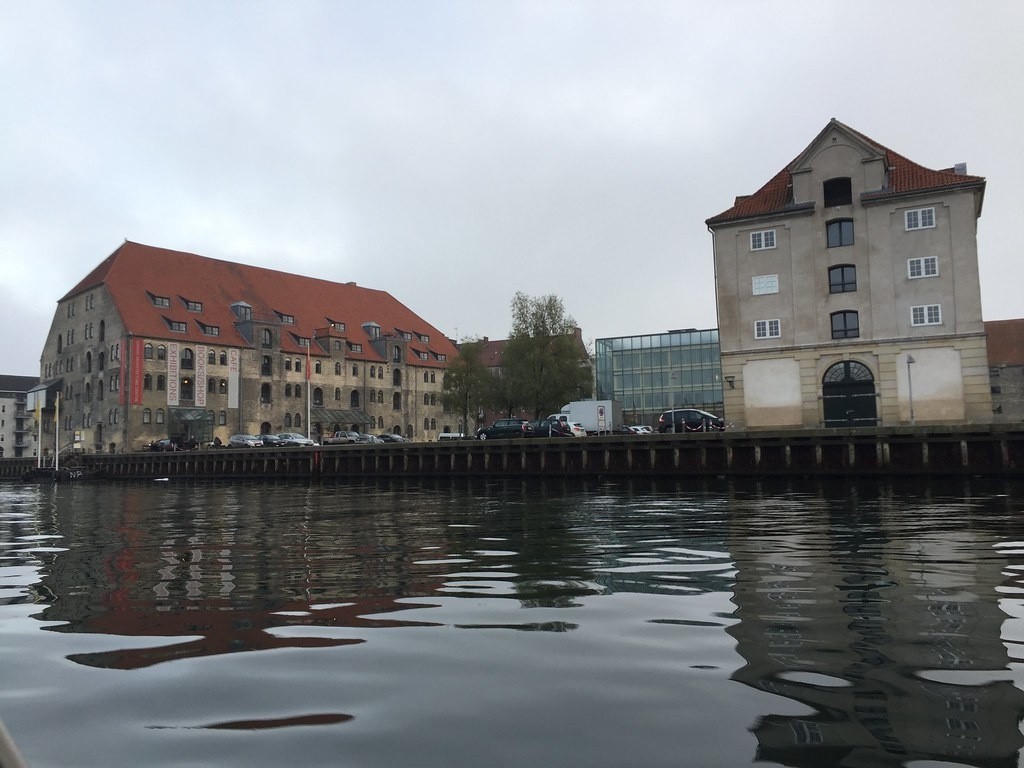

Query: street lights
[907,353,916,420]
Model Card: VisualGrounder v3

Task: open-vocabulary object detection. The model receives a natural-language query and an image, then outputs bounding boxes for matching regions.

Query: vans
[658,409,725,433]
[437,433,469,441]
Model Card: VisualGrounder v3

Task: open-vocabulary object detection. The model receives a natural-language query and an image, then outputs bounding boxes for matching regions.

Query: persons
[214,436,222,448]
[189,434,196,450]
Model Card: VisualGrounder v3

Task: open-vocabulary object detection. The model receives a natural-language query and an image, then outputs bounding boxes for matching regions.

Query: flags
[307,351,311,379]
[54,398,58,422]
[35,399,40,422]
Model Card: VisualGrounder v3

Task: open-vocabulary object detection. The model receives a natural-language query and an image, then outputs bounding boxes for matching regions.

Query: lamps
[724,376,735,389]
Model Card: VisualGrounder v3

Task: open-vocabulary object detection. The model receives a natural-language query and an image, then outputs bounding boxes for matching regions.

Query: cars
[325,429,411,443]
[624,425,658,434]
[229,432,314,446]
[150,437,186,451]
[475,419,588,438]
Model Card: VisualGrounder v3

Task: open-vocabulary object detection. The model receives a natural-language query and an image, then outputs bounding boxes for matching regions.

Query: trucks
[547,400,626,434]
